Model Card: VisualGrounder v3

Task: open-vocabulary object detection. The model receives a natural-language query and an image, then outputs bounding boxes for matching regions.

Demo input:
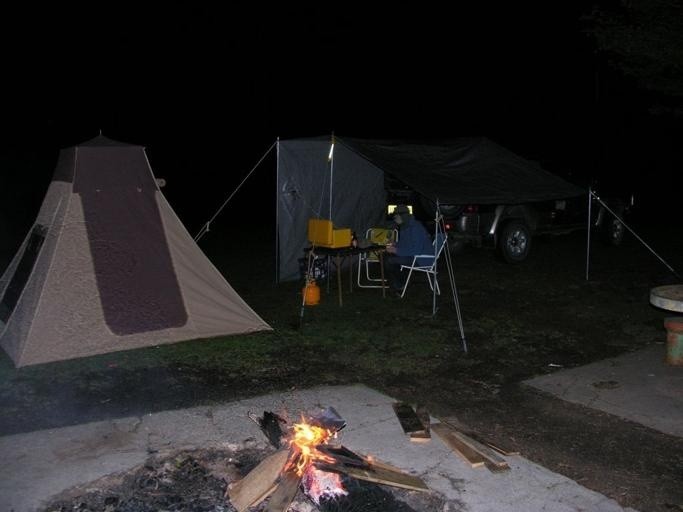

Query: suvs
[410,178,635,263]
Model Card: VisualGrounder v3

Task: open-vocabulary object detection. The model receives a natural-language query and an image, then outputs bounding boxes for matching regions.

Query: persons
[386,205,435,297]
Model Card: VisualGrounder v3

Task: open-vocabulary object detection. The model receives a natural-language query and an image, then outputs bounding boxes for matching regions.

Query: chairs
[400,233,448,314]
[357,228,399,290]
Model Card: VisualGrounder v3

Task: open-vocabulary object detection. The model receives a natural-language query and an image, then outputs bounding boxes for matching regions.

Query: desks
[650,285,683,313]
[302,248,388,308]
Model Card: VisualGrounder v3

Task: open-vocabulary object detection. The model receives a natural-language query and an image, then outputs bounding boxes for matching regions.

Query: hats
[389,205,411,217]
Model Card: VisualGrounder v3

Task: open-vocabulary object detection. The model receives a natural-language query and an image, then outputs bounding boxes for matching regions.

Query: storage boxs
[309,218,352,249]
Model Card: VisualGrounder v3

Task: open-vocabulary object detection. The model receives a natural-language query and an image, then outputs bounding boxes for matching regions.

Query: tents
[1,131,276,368]
